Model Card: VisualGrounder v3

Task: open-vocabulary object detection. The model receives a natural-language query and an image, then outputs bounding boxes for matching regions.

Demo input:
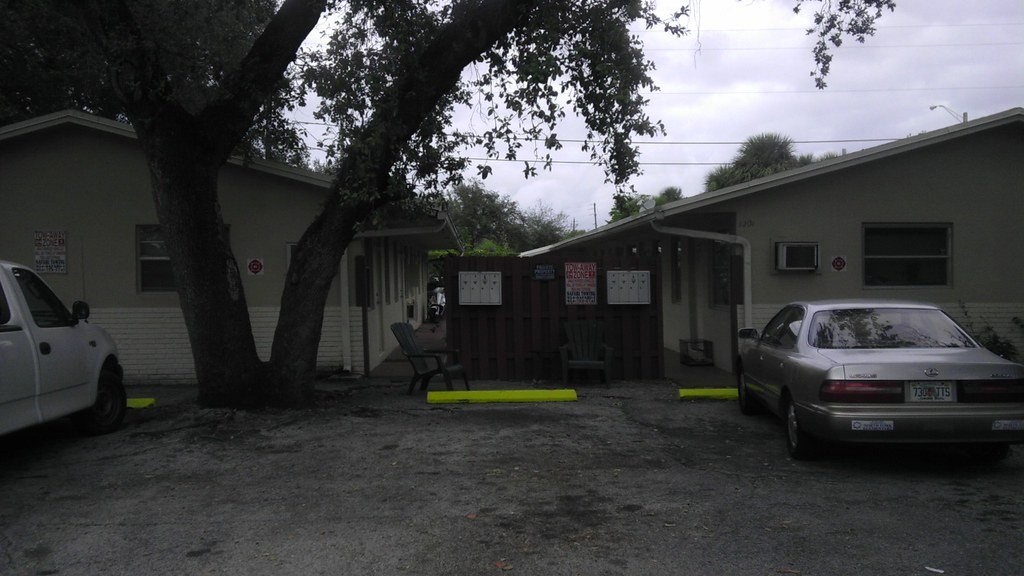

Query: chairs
[390,321,471,396]
[558,319,614,389]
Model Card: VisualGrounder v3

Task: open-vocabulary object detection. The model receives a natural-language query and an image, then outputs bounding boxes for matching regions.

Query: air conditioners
[776,242,818,270]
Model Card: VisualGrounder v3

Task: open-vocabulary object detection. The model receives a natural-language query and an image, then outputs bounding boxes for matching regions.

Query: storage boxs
[678,338,714,366]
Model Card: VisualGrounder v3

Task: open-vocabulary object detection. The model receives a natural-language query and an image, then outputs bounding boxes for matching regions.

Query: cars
[0,259,129,446]
[736,298,1024,462]
